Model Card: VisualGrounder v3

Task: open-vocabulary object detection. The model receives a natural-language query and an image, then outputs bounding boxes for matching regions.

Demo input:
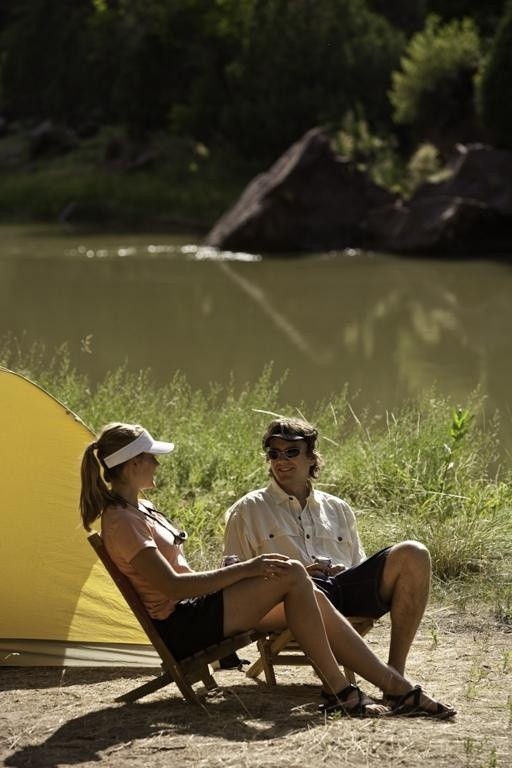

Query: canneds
[222,555,240,568]
[314,556,332,577]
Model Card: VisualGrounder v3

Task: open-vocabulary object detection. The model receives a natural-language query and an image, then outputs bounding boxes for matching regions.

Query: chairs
[86,530,268,720]
[245,615,375,688]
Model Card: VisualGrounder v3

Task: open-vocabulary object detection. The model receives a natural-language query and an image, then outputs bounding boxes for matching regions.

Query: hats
[102,428,175,469]
[262,427,304,448]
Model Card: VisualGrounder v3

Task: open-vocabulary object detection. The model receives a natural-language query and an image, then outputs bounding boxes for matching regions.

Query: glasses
[265,448,301,460]
[157,516,189,544]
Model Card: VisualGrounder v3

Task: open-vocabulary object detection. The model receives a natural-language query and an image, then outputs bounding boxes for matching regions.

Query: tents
[1,361,244,673]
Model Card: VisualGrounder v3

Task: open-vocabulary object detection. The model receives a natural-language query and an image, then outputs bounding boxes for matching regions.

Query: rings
[270,573,275,581]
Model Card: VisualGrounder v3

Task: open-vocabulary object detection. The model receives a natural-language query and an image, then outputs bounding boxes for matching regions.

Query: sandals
[320,684,395,718]
[382,685,457,721]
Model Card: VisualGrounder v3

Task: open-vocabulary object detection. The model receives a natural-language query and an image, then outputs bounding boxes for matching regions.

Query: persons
[222,417,431,701]
[79,422,457,719]
